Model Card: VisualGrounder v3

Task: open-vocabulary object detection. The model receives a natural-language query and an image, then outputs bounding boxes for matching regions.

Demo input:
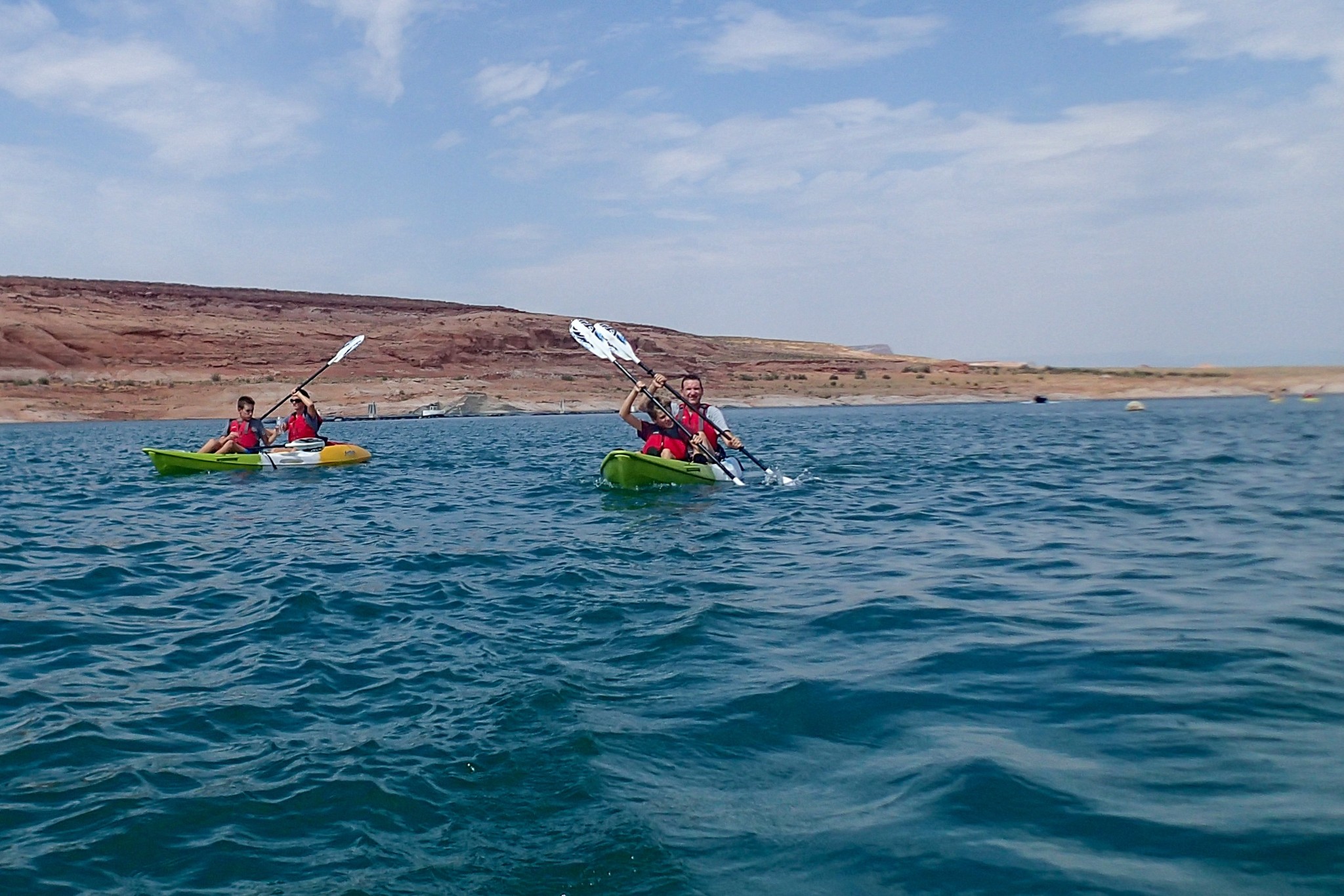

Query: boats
[1268,397,1284,404]
[142,437,372,476]
[1124,400,1146,411]
[1033,394,1048,404]
[598,447,746,490]
[419,404,446,419]
[1300,397,1321,403]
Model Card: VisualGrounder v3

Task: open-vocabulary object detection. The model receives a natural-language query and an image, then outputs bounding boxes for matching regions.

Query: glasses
[290,399,303,403]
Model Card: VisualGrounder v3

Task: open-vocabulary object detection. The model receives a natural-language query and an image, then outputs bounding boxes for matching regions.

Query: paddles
[166,437,325,453]
[593,321,796,487]
[261,334,364,421]
[568,317,746,487]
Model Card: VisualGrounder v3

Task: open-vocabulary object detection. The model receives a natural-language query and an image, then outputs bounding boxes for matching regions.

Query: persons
[619,381,715,462]
[638,373,742,464]
[197,395,284,454]
[265,389,323,453]
